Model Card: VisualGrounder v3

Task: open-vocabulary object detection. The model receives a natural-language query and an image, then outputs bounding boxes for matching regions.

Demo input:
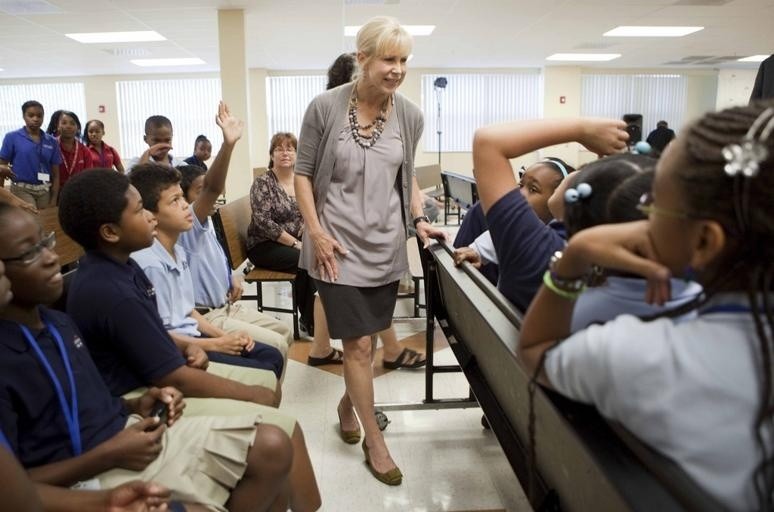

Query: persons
[453,155,572,269]
[177,99,293,388]
[46,109,94,186]
[0,204,294,511]
[304,50,429,370]
[293,14,451,486]
[123,115,179,177]
[0,162,40,215]
[567,165,704,333]
[246,131,318,337]
[453,195,499,285]
[0,257,213,511]
[175,135,213,172]
[471,115,630,316]
[56,167,324,512]
[128,160,285,381]
[84,119,126,176]
[647,120,675,150]
[564,153,659,241]
[1,99,64,209]
[515,100,773,511]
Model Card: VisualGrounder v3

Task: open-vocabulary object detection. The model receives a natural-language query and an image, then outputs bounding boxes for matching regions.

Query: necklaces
[348,83,394,148]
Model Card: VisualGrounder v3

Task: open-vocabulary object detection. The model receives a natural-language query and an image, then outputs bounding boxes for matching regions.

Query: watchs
[413,215,431,229]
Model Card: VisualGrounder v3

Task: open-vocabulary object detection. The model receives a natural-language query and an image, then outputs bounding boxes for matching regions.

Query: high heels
[337,406,361,443]
[362,433,403,486]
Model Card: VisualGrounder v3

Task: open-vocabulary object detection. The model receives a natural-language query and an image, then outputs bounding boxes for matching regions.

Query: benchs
[416,164,444,199]
[216,193,300,341]
[416,235,728,511]
[29,205,83,309]
[440,171,477,225]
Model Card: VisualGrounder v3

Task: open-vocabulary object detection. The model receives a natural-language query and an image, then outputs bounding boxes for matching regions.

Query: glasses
[637,190,693,220]
[274,146,296,153]
[0,231,57,265]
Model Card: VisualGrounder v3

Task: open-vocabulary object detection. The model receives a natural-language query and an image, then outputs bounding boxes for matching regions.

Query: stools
[406,236,426,318]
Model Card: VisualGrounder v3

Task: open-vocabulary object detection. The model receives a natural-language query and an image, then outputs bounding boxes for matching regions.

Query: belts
[11,181,52,191]
[196,307,214,315]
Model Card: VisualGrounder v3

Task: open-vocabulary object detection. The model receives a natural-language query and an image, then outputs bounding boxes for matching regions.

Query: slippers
[308,348,343,366]
[382,348,427,369]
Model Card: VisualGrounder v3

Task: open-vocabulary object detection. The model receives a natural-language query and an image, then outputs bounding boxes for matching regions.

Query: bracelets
[542,270,588,300]
[548,250,597,291]
